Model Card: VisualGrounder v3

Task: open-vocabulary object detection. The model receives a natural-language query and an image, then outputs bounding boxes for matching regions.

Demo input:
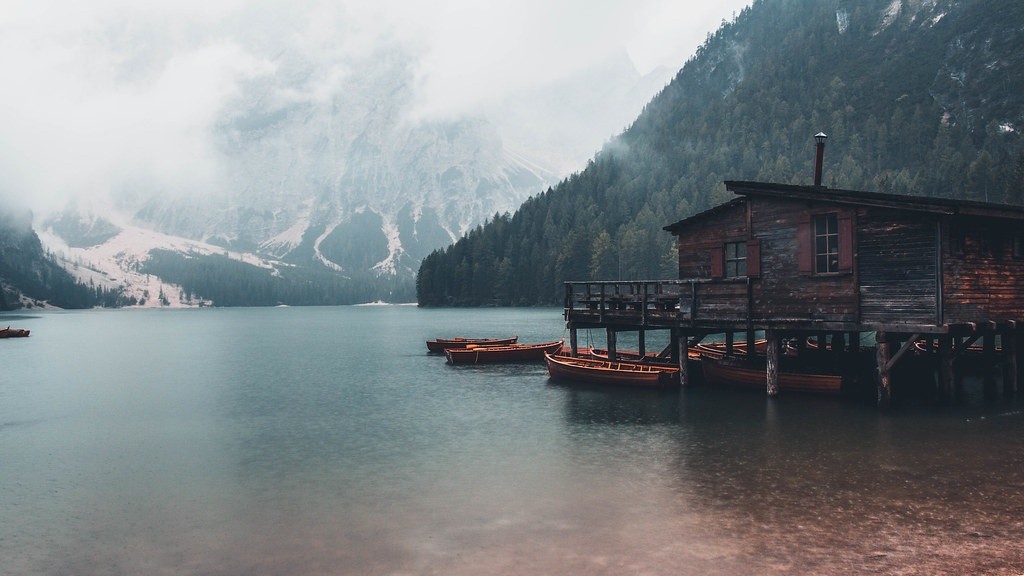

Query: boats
[426,335,519,352]
[443,338,565,364]
[913,339,1002,358]
[543,336,877,396]
